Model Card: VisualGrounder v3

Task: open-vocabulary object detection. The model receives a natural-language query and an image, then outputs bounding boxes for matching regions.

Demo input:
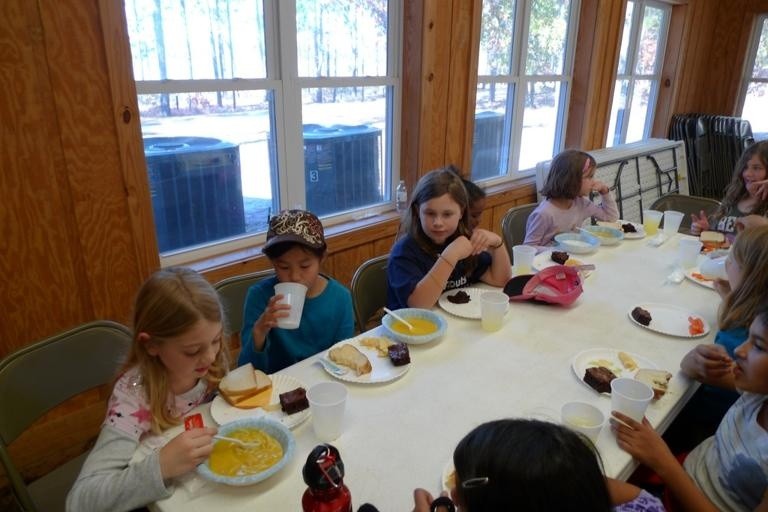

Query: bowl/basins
[380,307,448,346]
[553,232,602,255]
[193,417,297,487]
[581,224,624,247]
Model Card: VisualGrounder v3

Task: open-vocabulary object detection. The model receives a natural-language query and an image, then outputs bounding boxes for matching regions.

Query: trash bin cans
[143,136,246,253]
[266,123,382,218]
[471,111,506,181]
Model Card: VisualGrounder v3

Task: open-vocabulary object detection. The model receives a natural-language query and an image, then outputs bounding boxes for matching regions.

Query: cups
[560,402,605,450]
[662,211,685,240]
[274,282,309,329]
[513,245,537,276]
[642,208,662,237]
[305,380,347,443]
[607,375,656,431]
[476,290,509,332]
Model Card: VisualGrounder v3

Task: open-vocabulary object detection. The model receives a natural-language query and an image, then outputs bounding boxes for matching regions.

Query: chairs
[667,109,759,199]
[644,193,728,237]
[348,253,390,335]
[0,320,139,512]
[501,203,544,265]
[205,269,279,371]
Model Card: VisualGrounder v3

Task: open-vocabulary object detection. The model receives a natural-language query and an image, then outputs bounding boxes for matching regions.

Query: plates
[628,301,711,338]
[683,269,717,291]
[441,458,458,511]
[600,220,648,240]
[209,373,313,432]
[323,336,412,385]
[438,287,513,321]
[532,250,593,282]
[573,347,663,398]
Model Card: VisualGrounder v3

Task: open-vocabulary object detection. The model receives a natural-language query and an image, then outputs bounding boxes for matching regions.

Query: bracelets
[428,272,446,291]
[488,239,505,250]
[437,253,454,269]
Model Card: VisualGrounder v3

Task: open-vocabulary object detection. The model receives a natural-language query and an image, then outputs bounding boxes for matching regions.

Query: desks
[531,133,690,228]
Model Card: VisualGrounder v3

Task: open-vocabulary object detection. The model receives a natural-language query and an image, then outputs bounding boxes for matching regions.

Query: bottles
[300,440,351,512]
[396,179,408,213]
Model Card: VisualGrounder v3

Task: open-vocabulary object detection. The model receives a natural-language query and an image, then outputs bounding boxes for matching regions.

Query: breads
[219,362,272,408]
[634,368,673,400]
[699,231,730,249]
[329,343,372,375]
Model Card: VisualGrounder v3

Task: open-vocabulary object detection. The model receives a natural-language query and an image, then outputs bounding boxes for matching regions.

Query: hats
[503,262,596,309]
[261,209,324,253]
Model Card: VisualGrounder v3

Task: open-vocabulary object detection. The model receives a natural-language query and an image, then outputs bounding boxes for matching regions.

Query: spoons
[208,431,261,450]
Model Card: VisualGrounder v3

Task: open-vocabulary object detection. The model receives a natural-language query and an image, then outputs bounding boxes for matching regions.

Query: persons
[237,207,356,377]
[522,148,620,257]
[409,416,665,512]
[65,266,235,511]
[384,166,513,312]
[448,164,486,232]
